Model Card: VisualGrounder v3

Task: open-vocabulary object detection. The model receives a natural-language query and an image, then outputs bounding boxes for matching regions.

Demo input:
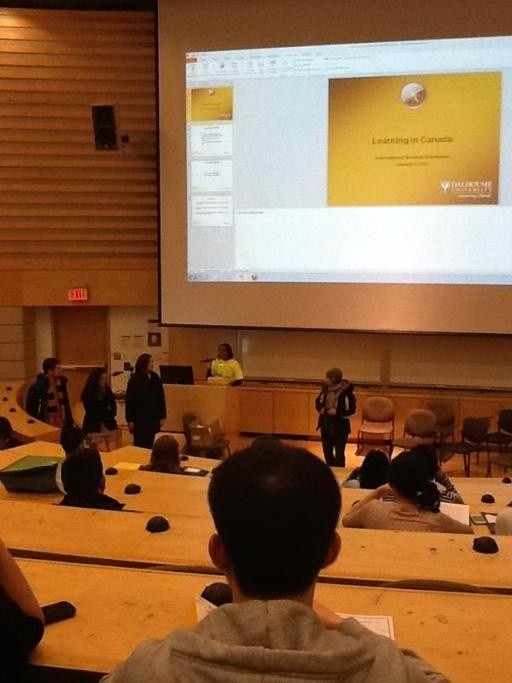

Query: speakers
[92,104,118,151]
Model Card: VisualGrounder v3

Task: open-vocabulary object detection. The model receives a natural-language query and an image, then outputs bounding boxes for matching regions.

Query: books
[433,500,470,526]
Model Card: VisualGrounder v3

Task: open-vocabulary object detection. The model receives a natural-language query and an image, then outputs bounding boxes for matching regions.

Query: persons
[341,447,389,490]
[342,450,474,534]
[97,438,447,682]
[58,426,106,499]
[25,358,73,428]
[206,343,244,386]
[79,367,118,442]
[410,443,463,504]
[141,435,188,476]
[0,537,48,683]
[1,416,22,450]
[124,352,168,450]
[313,366,357,467]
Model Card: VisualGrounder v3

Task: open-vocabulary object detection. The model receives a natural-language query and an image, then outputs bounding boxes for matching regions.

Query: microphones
[201,358,216,364]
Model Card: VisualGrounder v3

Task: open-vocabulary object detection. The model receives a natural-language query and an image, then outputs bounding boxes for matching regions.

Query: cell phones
[40,601,76,627]
[470,514,486,525]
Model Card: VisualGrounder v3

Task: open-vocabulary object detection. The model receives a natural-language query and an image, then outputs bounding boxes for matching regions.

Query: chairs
[425,401,455,466]
[477,408,512,472]
[183,412,231,460]
[439,417,489,477]
[356,396,395,459]
[389,409,437,460]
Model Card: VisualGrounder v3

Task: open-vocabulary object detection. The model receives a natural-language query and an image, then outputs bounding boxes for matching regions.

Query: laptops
[159,365,194,386]
